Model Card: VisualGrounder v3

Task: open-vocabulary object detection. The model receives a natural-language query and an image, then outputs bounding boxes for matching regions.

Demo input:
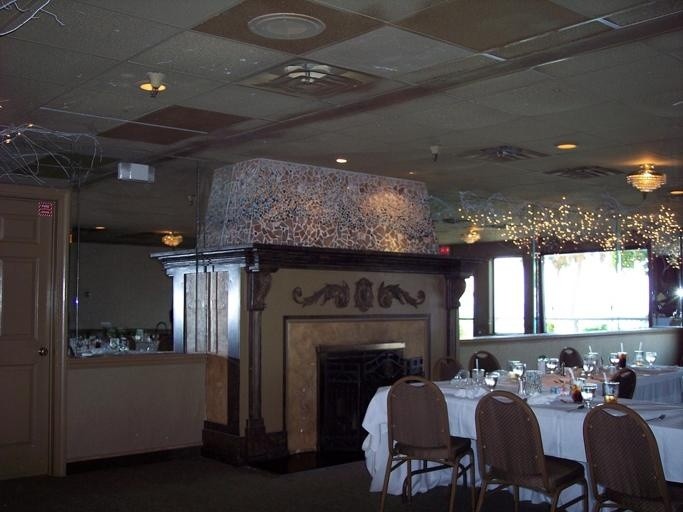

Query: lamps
[626,165,667,194]
[460,226,481,243]
[162,232,184,246]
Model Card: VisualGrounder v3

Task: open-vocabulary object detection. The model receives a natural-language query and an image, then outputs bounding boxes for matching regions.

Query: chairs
[379,376,474,512]
[582,402,682,512]
[475,391,589,512]
[559,346,583,368]
[603,367,636,399]
[468,351,501,377]
[432,356,464,381]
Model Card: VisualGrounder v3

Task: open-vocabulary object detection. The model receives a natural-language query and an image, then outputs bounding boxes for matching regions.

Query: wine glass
[645,352,657,369]
[582,356,597,381]
[512,361,529,399]
[456,369,498,393]
[608,353,619,367]
[581,383,595,412]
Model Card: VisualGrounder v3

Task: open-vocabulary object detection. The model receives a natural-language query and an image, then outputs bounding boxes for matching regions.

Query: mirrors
[67,229,207,358]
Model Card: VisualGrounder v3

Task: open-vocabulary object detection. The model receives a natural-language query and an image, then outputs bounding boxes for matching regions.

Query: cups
[617,352,628,368]
[571,380,584,403]
[588,352,601,375]
[602,382,619,403]
[545,358,558,376]
[634,351,644,365]
[110,337,119,350]
